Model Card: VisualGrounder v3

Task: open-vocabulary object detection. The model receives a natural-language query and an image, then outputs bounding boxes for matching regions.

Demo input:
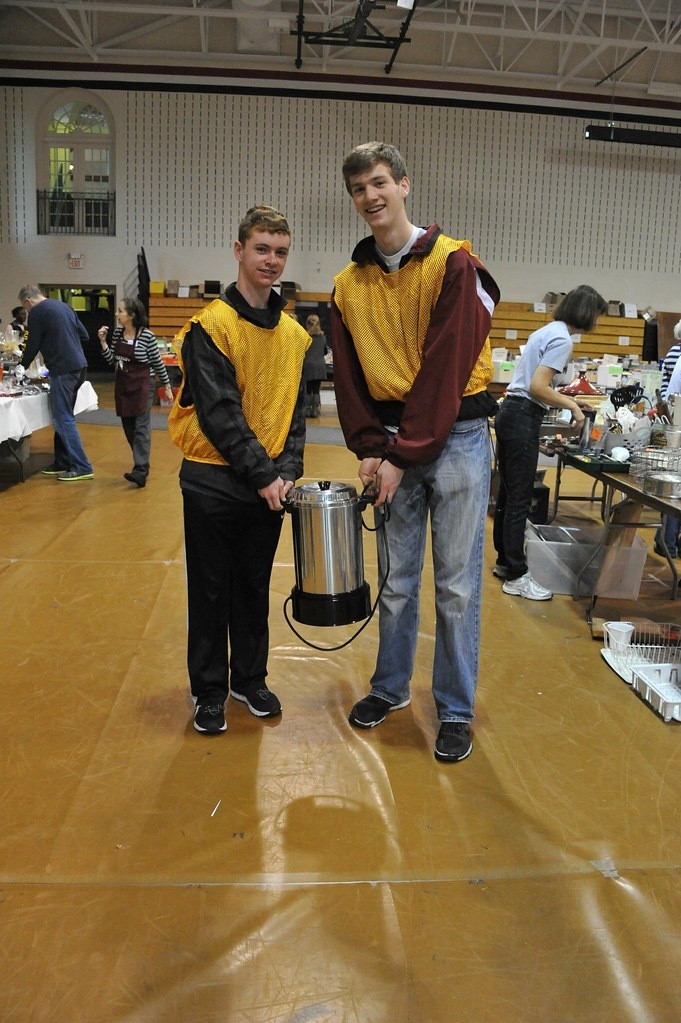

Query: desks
[0,379,98,494]
[488,420,573,475]
[163,363,179,367]
[542,448,681,626]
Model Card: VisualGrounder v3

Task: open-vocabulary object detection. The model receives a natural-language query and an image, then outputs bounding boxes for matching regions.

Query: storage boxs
[602,621,681,675]
[149,280,166,293]
[556,354,664,396]
[547,303,559,313]
[489,359,515,384]
[630,443,681,486]
[528,523,648,602]
[198,280,224,299]
[167,280,181,295]
[530,302,547,313]
[190,289,202,297]
[158,386,179,407]
[566,450,630,474]
[177,286,190,297]
[620,302,638,319]
[629,662,681,722]
[607,300,621,317]
[528,480,550,525]
[160,353,178,364]
[157,343,172,351]
[541,291,557,304]
[556,292,566,303]
[576,394,608,406]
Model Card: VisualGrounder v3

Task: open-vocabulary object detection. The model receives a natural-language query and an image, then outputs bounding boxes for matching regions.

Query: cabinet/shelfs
[146,292,646,361]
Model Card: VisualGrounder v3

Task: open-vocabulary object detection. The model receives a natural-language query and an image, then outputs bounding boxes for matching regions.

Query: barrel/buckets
[281,480,378,626]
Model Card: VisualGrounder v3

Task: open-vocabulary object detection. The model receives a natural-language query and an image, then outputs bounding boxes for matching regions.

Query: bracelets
[165,385,171,390]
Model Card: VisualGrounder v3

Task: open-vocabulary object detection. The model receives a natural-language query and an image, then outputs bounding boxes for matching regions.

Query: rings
[172,397,173,399]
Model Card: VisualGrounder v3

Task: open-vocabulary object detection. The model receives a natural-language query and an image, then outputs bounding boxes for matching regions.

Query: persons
[5,307,28,351]
[492,285,610,601]
[13,284,94,481]
[98,297,175,487]
[305,314,329,418]
[167,206,312,731]
[332,141,502,763]
[652,319,681,558]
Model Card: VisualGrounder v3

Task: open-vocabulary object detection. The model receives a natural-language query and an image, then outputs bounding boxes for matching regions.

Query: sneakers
[229,685,283,717]
[348,694,410,729]
[493,567,553,601]
[433,720,473,762]
[189,688,230,734]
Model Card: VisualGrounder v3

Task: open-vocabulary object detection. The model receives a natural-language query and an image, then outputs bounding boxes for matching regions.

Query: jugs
[569,409,597,454]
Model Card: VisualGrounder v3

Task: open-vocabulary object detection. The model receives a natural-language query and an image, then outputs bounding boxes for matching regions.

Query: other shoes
[653,543,676,559]
[40,464,93,481]
[123,471,145,488]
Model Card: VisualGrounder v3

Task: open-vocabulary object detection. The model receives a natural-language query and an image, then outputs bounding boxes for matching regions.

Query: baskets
[629,444,680,483]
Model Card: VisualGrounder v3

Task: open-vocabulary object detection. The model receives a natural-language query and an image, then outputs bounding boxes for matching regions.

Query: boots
[306,394,319,417]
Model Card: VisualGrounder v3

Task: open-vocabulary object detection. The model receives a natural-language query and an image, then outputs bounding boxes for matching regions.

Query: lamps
[584,46,681,148]
